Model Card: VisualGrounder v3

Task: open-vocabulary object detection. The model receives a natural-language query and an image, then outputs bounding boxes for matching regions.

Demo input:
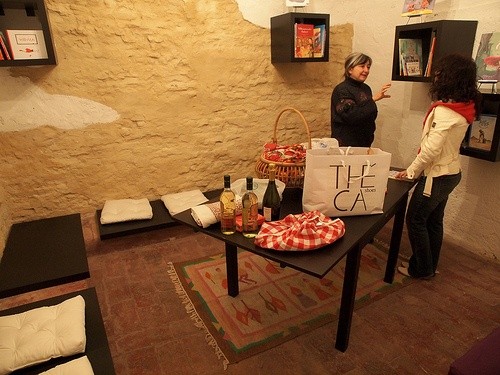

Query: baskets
[255,107,311,188]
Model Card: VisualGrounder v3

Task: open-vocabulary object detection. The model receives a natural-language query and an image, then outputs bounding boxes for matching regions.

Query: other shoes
[401,262,409,268]
[398,266,412,278]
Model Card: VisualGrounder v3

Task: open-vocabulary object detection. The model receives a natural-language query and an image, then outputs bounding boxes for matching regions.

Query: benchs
[0,287,115,375]
[96,188,224,239]
[0,213,91,298]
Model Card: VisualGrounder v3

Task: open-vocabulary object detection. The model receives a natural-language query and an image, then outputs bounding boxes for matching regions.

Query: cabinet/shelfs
[0,0,58,67]
[270,12,330,65]
[391,20,479,82]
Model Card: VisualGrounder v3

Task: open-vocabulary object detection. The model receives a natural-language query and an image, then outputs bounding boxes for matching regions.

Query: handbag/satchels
[302,146,392,217]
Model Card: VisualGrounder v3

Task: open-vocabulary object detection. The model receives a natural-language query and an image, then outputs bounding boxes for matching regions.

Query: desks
[172,165,419,353]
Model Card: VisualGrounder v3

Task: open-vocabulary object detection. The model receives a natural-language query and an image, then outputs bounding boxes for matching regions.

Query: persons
[395,53,480,279]
[298,38,313,58]
[329,51,392,147]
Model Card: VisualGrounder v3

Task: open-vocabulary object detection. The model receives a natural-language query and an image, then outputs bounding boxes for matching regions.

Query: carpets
[168,237,419,369]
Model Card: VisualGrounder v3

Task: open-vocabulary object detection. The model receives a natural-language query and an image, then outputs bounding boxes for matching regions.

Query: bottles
[242,176,260,239]
[219,174,237,235]
[262,163,280,222]
